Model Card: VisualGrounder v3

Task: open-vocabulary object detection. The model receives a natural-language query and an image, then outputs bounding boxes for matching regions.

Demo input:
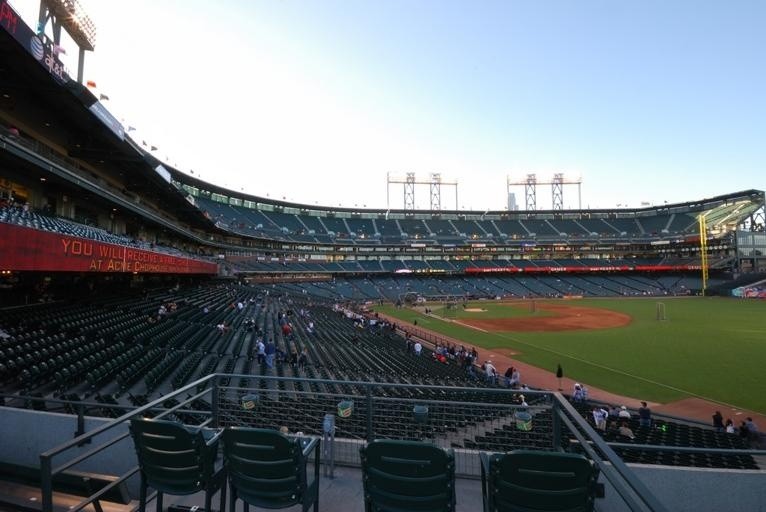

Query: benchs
[1,459,134,512]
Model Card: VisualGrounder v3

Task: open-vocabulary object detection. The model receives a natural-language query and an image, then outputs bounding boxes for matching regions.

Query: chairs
[0,301,544,454]
[225,423,320,512]
[2,193,763,302]
[480,449,597,512]
[542,389,766,469]
[127,415,229,512]
[358,439,458,512]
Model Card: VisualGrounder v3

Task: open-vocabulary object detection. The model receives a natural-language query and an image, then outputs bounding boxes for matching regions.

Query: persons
[190,190,765,305]
[159,305,315,362]
[432,340,528,389]
[332,306,421,355]
[516,364,758,438]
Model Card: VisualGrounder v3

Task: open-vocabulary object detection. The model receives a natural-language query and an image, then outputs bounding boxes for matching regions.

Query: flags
[52,41,202,175]
